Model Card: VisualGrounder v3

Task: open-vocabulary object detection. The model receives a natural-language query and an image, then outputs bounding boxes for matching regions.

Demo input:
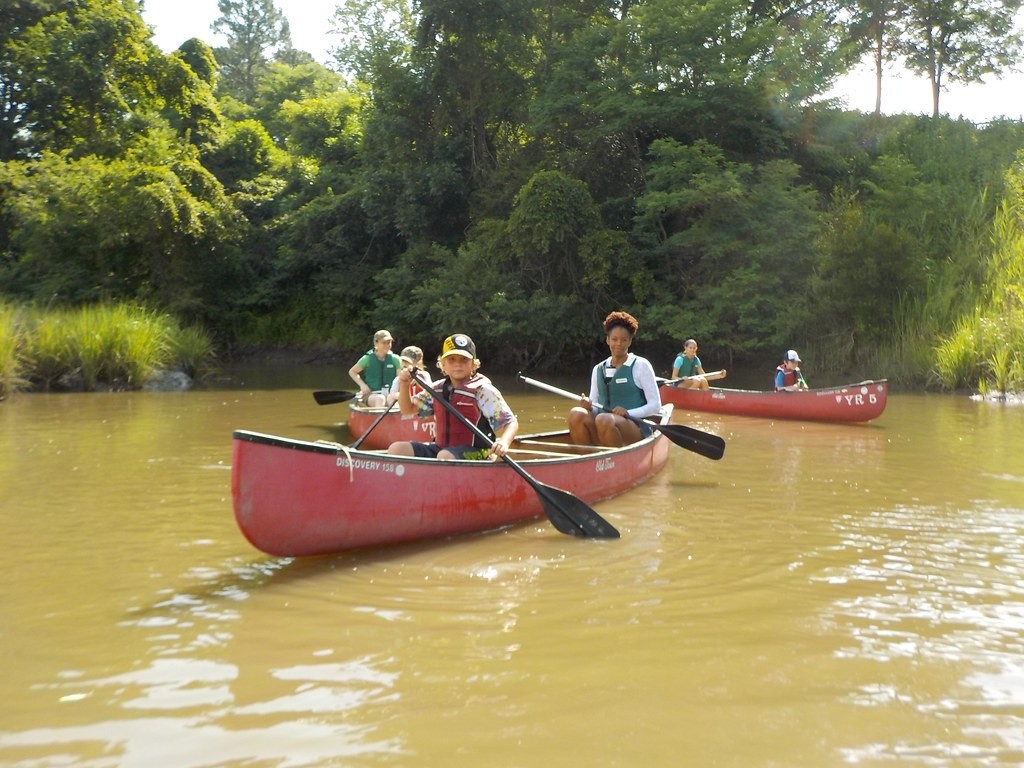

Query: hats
[374,330,394,343]
[400,345,424,372]
[441,333,476,362]
[784,350,801,363]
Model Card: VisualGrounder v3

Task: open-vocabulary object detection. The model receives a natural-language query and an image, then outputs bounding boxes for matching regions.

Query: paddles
[517,371,729,462]
[312,389,391,406]
[656,371,722,388]
[346,399,397,450]
[408,366,618,539]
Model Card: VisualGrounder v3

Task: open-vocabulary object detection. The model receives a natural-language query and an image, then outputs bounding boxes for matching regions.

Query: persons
[568,311,661,447]
[672,339,710,391]
[773,349,809,391]
[349,330,519,461]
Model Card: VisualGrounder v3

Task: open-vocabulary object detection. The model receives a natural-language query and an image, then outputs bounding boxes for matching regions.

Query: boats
[230,403,674,557]
[348,389,436,450]
[655,376,887,422]
